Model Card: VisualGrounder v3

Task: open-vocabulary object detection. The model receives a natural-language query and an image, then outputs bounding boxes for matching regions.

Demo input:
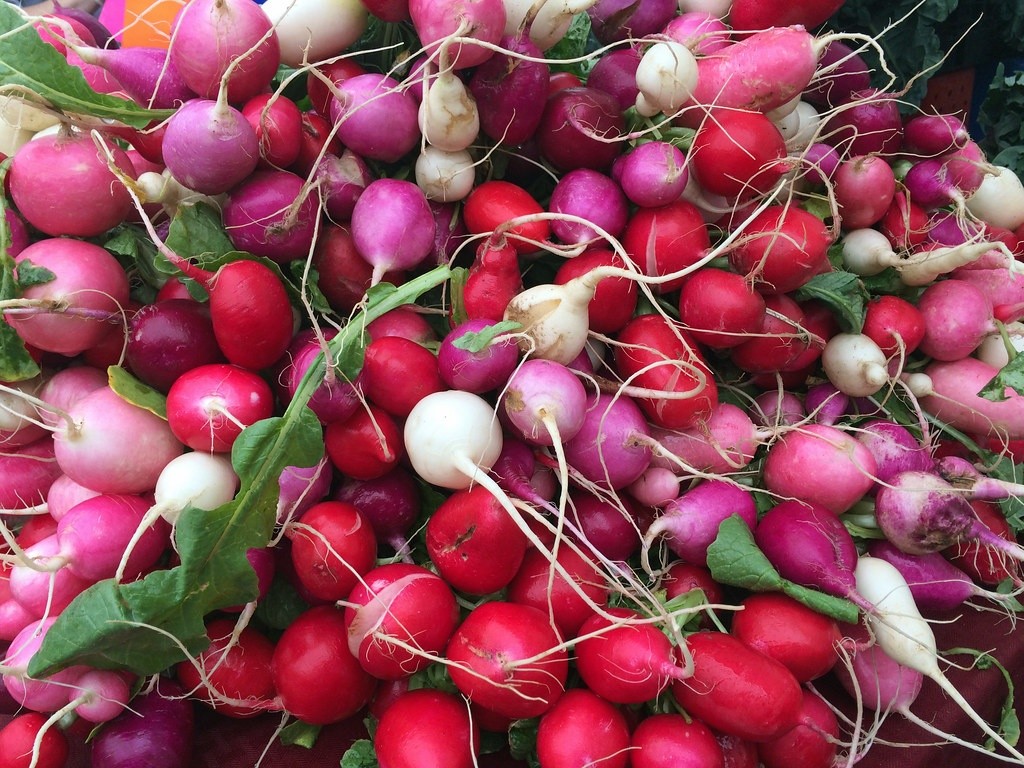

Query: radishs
[1,1,1024,680]
